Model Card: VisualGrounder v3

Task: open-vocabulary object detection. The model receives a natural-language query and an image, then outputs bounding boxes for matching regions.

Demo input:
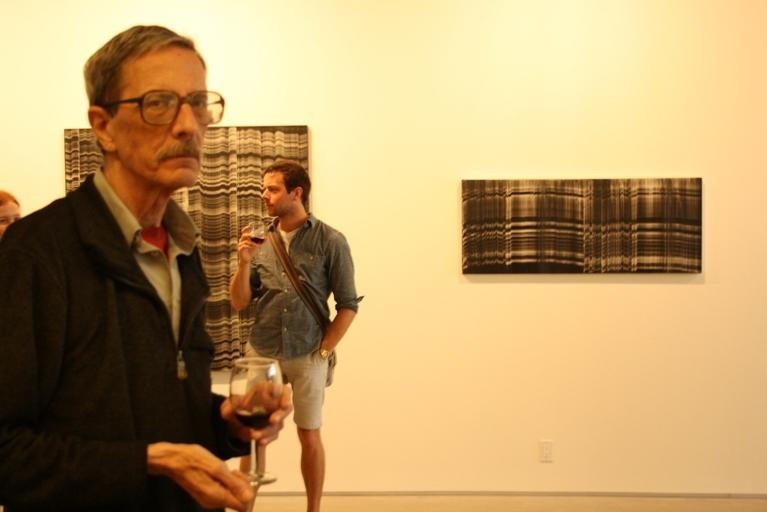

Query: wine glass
[228,355,285,487]
[249,219,269,264]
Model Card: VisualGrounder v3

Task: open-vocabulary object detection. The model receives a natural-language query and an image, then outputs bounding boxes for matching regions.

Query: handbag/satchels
[326,350,336,386]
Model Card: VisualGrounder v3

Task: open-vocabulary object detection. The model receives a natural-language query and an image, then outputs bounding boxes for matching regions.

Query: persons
[0,25,293,511]
[230,163,364,512]
[1,191,22,238]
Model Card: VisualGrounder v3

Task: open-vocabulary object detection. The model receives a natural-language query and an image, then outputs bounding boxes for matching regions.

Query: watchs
[318,349,329,359]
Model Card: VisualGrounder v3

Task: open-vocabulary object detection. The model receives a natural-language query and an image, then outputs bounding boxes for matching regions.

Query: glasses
[103,90,224,126]
